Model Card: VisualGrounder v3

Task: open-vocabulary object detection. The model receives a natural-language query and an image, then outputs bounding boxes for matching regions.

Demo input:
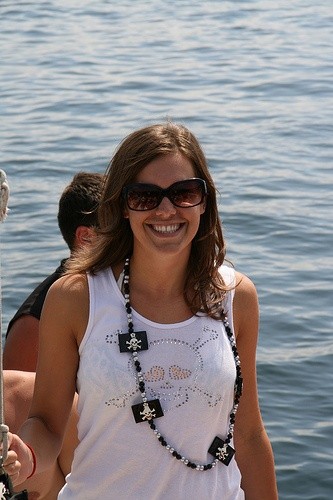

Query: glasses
[122,177,208,212]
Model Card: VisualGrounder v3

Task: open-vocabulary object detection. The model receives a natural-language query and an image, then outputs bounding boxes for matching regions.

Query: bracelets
[24,443,38,480]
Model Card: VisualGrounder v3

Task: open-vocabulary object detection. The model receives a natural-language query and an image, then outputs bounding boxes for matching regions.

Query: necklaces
[118,247,244,472]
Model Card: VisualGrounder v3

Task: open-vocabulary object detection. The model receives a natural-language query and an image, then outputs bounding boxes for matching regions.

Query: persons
[0,369,85,500]
[4,172,108,372]
[0,121,278,500]
